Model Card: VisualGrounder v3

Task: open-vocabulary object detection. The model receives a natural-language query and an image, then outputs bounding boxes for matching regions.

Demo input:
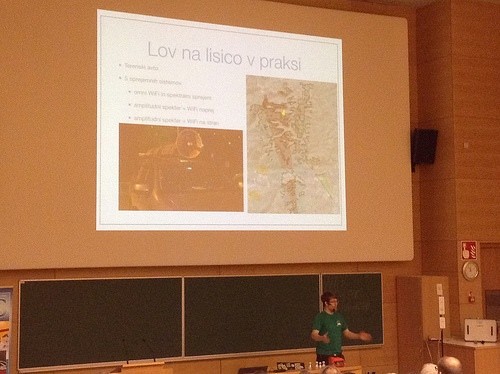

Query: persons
[421,362,441,374]
[434,357,463,374]
[311,291,372,366]
[321,366,340,374]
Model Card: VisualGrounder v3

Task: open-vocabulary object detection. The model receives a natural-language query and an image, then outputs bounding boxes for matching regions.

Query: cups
[474,339,477,344]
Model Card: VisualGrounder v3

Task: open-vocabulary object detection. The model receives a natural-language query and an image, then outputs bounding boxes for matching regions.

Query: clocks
[462,261,479,282]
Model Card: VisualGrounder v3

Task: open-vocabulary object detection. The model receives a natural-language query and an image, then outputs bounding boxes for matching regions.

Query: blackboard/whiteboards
[18,276,183,371]
[323,272,383,347]
[184,274,322,359]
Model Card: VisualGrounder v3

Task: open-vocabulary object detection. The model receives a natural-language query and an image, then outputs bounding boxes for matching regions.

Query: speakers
[412,128,438,163]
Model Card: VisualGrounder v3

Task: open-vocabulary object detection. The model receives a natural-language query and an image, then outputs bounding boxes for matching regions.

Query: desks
[424,335,500,374]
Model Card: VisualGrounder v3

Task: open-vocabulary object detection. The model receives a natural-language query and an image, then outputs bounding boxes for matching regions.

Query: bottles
[316,361,326,368]
[307,362,312,369]
